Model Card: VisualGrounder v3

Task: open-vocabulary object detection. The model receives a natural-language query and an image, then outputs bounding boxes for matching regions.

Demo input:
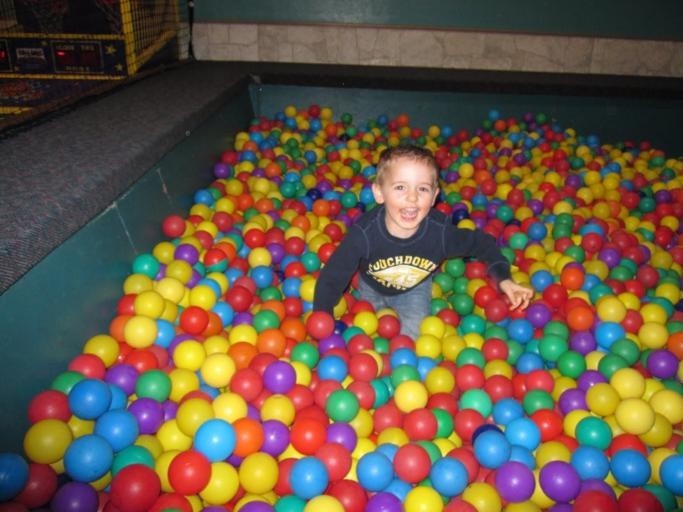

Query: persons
[312,147,534,343]
